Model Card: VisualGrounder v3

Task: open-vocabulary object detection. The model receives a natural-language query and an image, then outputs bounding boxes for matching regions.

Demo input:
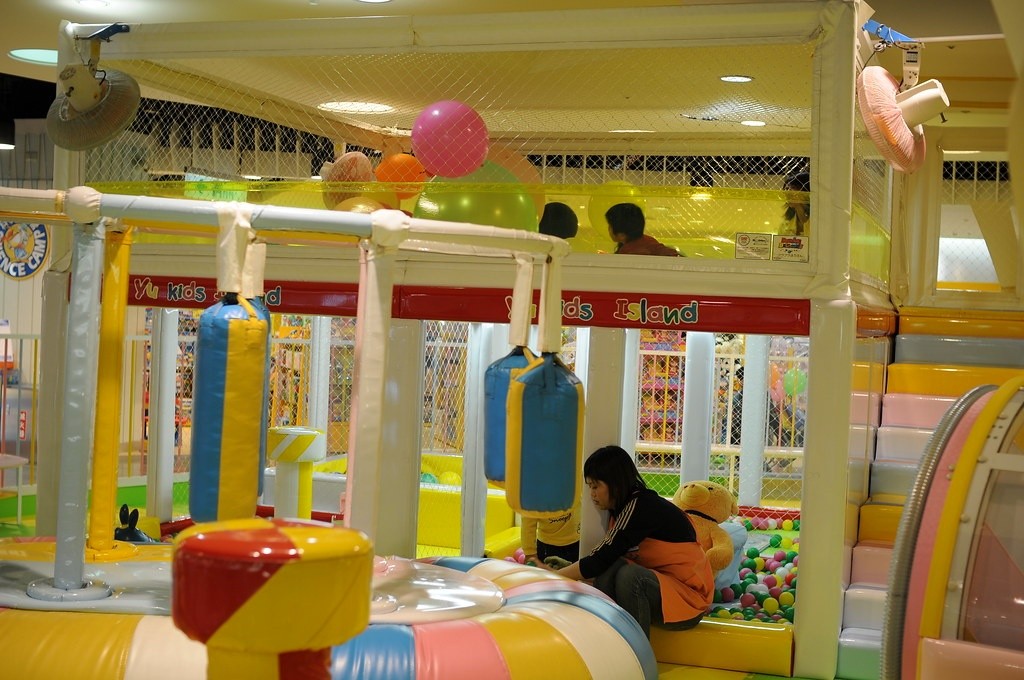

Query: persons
[721,366,780,477]
[781,173,811,237]
[527,444,714,642]
[605,202,684,256]
[520,481,581,570]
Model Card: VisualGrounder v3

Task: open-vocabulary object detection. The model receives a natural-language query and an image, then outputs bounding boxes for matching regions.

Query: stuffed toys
[637,330,745,443]
[671,480,739,583]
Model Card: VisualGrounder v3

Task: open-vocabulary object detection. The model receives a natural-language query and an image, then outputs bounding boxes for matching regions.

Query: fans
[46,22,141,150]
[857,20,949,174]
